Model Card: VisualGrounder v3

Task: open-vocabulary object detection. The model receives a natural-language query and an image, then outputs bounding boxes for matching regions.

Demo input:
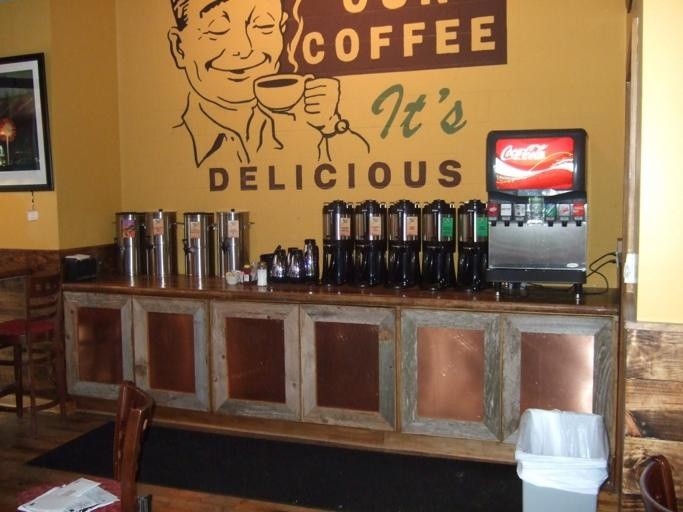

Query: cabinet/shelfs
[214,295,397,450]
[58,290,212,436]
[397,304,619,484]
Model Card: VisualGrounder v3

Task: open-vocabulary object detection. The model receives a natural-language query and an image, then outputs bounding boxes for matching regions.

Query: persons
[167,0,371,169]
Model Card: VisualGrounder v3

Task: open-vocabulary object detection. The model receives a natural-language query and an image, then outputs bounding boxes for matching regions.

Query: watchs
[318,119,349,139]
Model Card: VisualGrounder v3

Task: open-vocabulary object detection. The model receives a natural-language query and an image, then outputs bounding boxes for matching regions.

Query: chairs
[634,453,682,511]
[17,380,154,512]
[0,273,66,436]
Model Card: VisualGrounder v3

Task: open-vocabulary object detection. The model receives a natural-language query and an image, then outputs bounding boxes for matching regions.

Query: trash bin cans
[514,407,609,512]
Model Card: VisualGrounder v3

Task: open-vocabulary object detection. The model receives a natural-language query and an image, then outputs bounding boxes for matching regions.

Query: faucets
[182,238,191,254]
[219,239,227,255]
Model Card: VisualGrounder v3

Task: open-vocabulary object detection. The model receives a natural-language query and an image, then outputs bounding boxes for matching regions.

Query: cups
[259,253,273,270]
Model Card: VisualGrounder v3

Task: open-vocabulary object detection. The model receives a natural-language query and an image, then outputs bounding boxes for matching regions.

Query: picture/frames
[0,51,53,194]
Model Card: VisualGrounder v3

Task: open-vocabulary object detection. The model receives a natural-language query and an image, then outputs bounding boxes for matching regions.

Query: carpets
[24,418,523,512]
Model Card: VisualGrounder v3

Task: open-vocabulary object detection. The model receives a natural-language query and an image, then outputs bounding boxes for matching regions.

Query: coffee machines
[353,199,386,289]
[387,198,421,291]
[421,198,457,293]
[320,200,353,286]
[455,200,487,291]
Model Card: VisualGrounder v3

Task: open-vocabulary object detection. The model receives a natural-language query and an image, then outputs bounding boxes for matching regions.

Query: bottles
[242,265,252,286]
[256,262,267,288]
[268,239,318,283]
